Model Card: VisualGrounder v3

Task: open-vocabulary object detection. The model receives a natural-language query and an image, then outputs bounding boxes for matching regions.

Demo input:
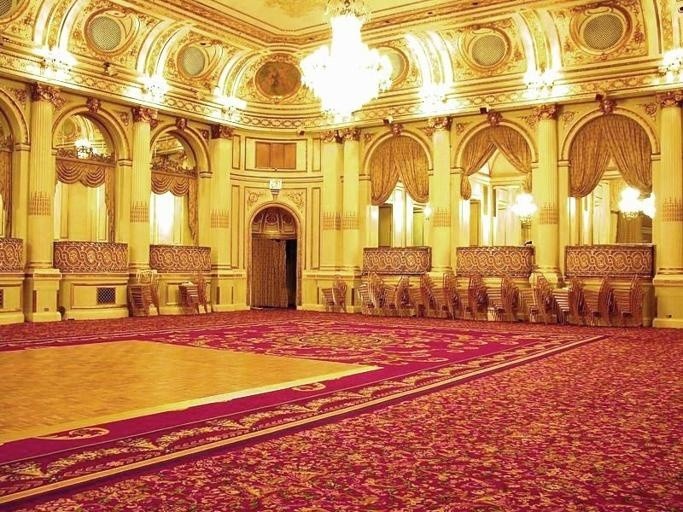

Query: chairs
[176,266,213,317]
[127,268,161,317]
